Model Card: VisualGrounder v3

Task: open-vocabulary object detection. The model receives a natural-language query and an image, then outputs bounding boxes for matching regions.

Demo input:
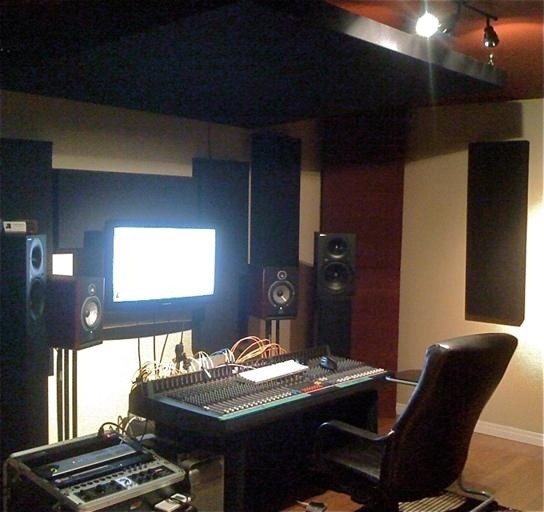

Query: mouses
[320,356,337,369]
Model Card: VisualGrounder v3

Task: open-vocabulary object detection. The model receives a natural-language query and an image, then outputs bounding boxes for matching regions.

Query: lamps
[413,0,499,48]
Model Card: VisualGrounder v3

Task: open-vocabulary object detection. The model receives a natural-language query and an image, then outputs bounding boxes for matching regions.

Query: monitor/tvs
[104,218,216,303]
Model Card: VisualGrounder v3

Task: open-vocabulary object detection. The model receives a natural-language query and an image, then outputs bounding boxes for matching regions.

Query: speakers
[49,274,106,350]
[312,232,357,355]
[247,263,299,320]
[0,233,54,376]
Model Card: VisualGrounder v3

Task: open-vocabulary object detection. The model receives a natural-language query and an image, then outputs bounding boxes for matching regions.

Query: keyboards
[239,359,310,383]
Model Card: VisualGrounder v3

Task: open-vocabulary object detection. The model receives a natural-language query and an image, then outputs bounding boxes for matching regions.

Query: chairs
[291,332,518,512]
